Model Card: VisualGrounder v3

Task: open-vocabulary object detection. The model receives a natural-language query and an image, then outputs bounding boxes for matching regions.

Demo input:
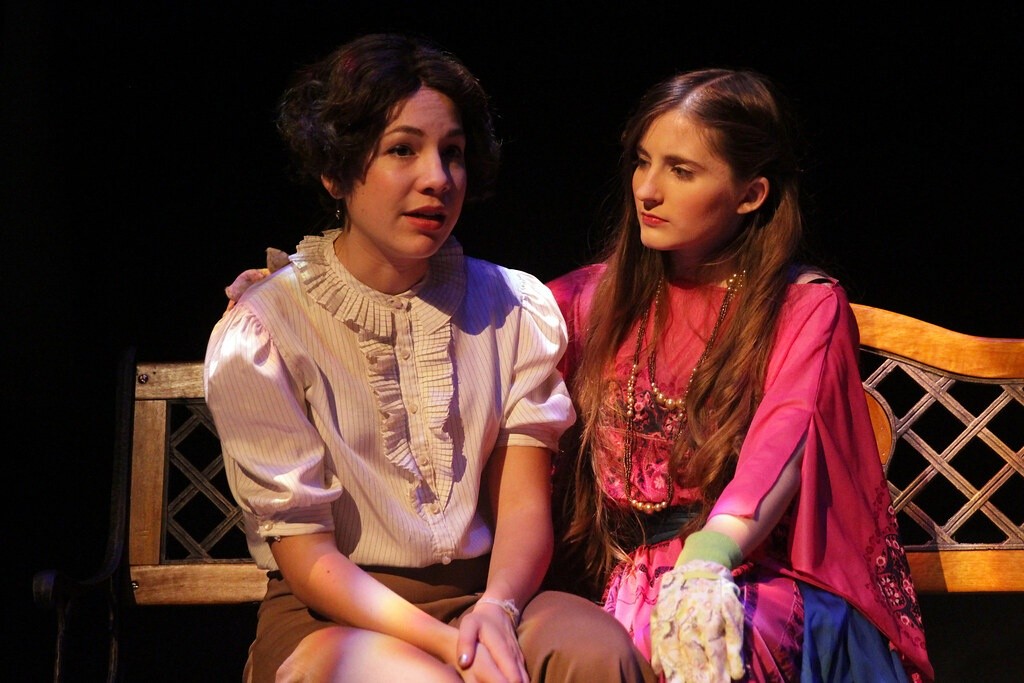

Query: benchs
[117,304,1024,683]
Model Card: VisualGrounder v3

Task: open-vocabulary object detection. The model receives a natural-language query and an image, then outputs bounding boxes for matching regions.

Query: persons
[201,35,657,683]
[224,65,935,683]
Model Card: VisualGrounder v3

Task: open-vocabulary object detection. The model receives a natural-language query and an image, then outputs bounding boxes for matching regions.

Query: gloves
[651,530,745,683]
[222,248,291,319]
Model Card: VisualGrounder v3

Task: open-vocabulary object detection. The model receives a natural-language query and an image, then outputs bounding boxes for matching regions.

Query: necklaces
[621,272,746,513]
